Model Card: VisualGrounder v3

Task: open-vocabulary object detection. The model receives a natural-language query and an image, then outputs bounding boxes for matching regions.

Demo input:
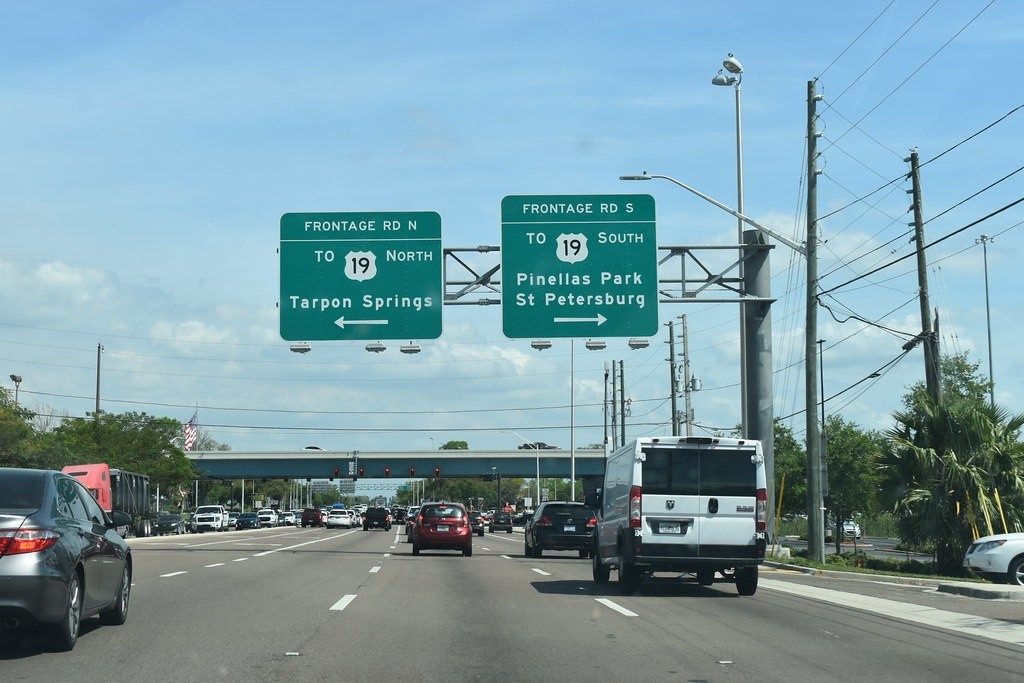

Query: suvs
[467,511,484,538]
[523,501,597,560]
[488,513,513,532]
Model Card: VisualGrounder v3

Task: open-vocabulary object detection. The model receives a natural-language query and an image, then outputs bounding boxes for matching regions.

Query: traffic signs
[501,194,657,337]
[278,211,443,341]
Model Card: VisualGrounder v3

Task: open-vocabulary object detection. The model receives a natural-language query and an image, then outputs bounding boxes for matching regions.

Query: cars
[409,502,475,556]
[828,518,862,541]
[0,467,134,650]
[301,445,327,452]
[481,508,534,525]
[230,512,238,526]
[579,445,605,449]
[151,511,193,536]
[257,503,420,532]
[962,530,1024,587]
[236,512,262,529]
[517,442,559,450]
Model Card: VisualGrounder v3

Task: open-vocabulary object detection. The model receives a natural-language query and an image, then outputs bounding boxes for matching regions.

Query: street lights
[975,233,995,408]
[501,430,540,507]
[711,55,750,438]
[9,374,23,407]
[621,175,821,561]
[817,339,826,429]
[430,436,434,447]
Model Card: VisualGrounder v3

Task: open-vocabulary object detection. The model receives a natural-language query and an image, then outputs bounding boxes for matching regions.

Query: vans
[587,437,769,596]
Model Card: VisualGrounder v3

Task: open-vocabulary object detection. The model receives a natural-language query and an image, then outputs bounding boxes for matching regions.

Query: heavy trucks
[61,463,152,538]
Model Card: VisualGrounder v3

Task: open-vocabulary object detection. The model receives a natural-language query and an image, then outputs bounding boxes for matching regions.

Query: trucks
[374,497,386,506]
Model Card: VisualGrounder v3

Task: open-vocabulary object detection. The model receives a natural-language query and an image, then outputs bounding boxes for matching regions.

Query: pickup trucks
[193,505,229,532]
